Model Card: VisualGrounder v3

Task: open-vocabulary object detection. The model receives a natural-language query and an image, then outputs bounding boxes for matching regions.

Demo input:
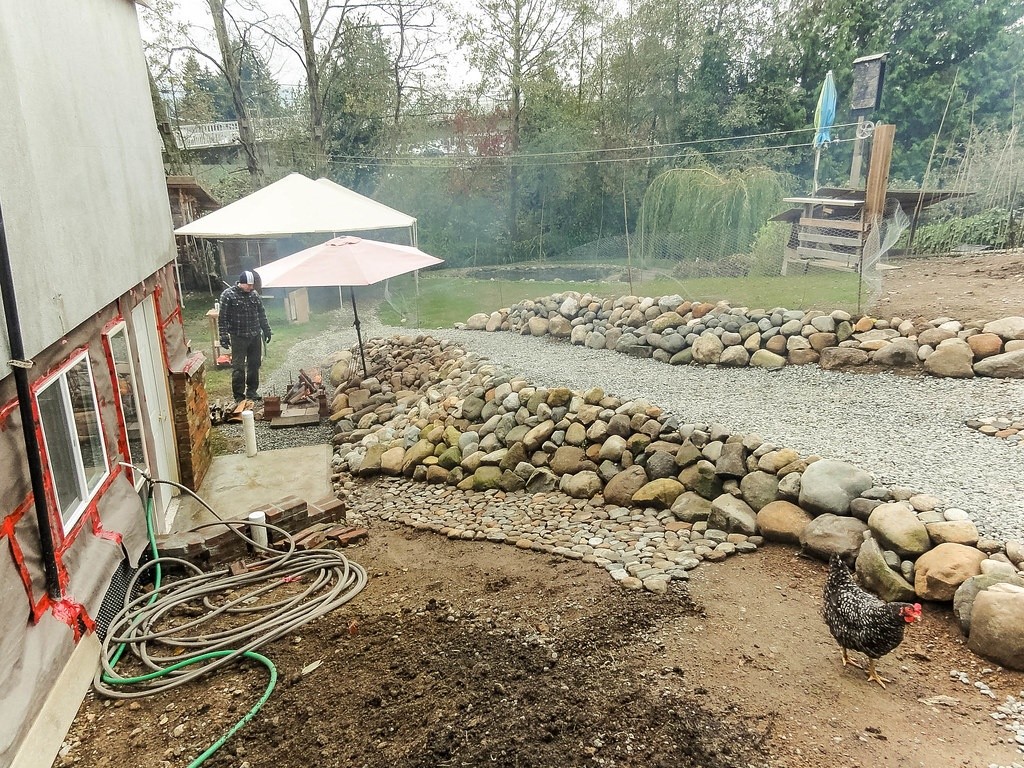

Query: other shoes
[246,391,262,400]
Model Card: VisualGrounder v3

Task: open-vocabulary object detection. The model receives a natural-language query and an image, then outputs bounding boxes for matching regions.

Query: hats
[239,271,255,285]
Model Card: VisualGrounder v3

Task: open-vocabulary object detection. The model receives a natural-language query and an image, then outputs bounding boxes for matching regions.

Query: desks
[206,309,264,370]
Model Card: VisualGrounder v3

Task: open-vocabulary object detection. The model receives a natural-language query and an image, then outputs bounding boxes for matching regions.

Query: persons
[218,270,271,403]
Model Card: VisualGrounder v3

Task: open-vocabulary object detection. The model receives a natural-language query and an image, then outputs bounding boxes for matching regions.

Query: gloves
[263,327,271,343]
[220,338,231,350]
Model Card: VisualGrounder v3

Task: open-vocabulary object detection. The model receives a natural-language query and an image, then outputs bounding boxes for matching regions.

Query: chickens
[822,549,922,690]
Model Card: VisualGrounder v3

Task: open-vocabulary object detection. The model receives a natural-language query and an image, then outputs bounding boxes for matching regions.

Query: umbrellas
[173,171,417,309]
[253,235,446,380]
[804,70,837,248]
